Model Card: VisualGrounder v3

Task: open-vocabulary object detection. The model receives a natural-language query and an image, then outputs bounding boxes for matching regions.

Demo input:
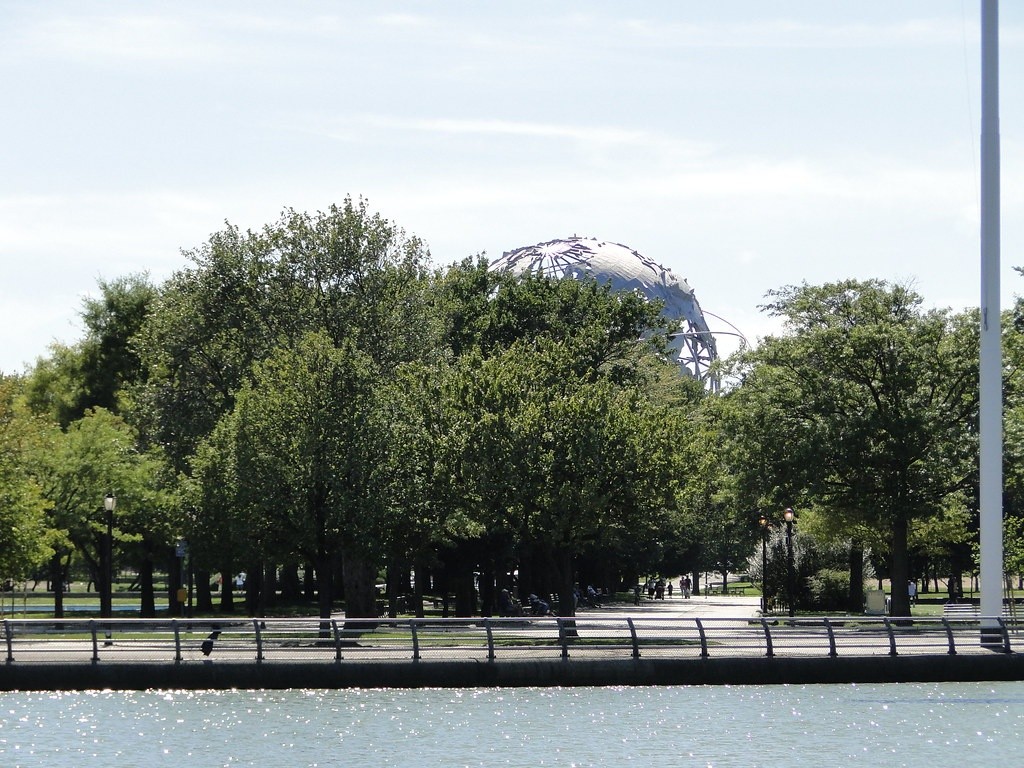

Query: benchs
[378,595,410,618]
[943,603,1024,631]
[550,593,559,612]
[708,586,719,595]
[517,598,535,616]
[725,587,745,597]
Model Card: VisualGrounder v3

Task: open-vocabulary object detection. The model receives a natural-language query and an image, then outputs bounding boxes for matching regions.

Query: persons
[646,576,692,601]
[634,586,642,606]
[500,589,550,617]
[946,578,959,603]
[573,582,603,610]
[908,580,916,608]
[234,574,245,591]
[216,576,222,592]
